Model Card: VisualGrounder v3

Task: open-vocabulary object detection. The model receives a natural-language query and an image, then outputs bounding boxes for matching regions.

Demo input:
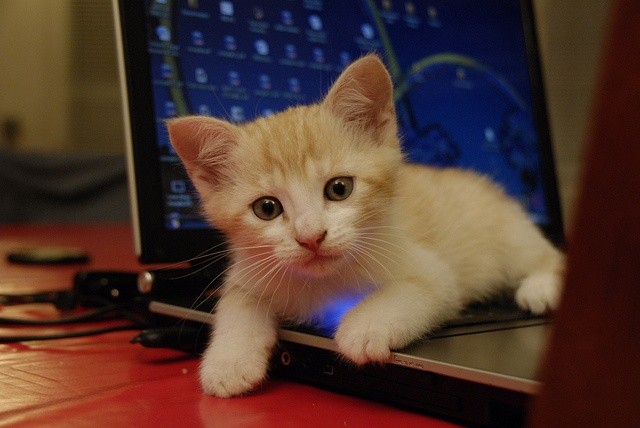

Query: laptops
[112,0,569,427]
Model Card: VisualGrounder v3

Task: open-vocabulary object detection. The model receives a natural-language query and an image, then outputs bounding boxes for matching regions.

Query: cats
[162,49,566,398]
